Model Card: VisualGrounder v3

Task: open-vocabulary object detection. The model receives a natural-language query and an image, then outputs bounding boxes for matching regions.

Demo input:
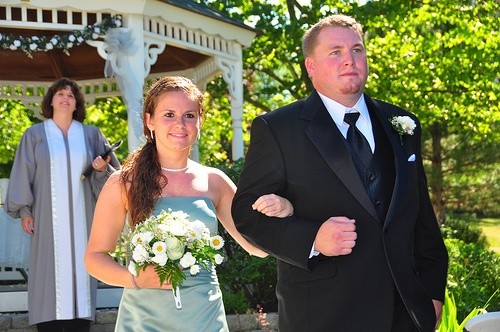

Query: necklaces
[157,158,192,172]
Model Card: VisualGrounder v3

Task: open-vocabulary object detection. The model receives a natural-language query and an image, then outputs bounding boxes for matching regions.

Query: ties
[343,111,383,211]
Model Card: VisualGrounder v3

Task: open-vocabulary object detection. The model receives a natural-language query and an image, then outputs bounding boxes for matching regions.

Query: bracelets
[130,273,139,289]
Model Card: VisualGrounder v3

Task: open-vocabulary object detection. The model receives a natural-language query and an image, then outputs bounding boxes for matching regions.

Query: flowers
[0,16,122,54]
[104,207,228,310]
[388,115,417,145]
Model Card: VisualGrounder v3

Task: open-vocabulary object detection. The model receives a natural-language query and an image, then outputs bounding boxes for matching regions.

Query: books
[80,140,124,181]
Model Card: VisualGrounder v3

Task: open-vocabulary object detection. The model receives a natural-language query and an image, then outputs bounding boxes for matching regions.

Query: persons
[84,75,294,332]
[230,12,449,332]
[4,77,122,332]
